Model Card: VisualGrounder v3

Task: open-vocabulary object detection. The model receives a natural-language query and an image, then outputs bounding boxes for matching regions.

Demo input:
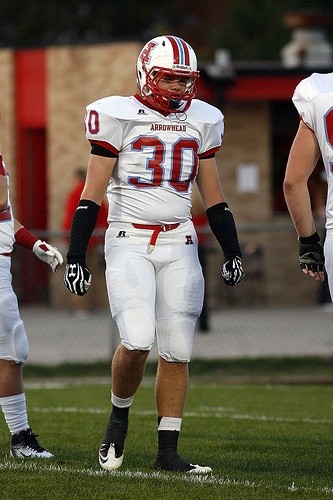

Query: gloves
[298,231,325,273]
[221,257,246,286]
[63,262,92,296]
[33,239,64,272]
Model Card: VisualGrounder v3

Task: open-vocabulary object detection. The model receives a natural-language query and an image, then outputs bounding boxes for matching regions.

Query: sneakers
[154,450,213,475]
[9,427,55,460]
[98,413,129,470]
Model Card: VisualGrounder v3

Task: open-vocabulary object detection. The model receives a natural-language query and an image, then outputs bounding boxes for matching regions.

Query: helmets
[135,35,199,100]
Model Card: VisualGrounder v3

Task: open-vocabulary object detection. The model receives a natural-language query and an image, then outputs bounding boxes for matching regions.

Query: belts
[133,223,180,253]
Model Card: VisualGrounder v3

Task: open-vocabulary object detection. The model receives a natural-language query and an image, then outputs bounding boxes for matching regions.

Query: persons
[59,168,211,335]
[61,35,246,473]
[310,155,332,305]
[0,152,63,459]
[283,72,333,305]
